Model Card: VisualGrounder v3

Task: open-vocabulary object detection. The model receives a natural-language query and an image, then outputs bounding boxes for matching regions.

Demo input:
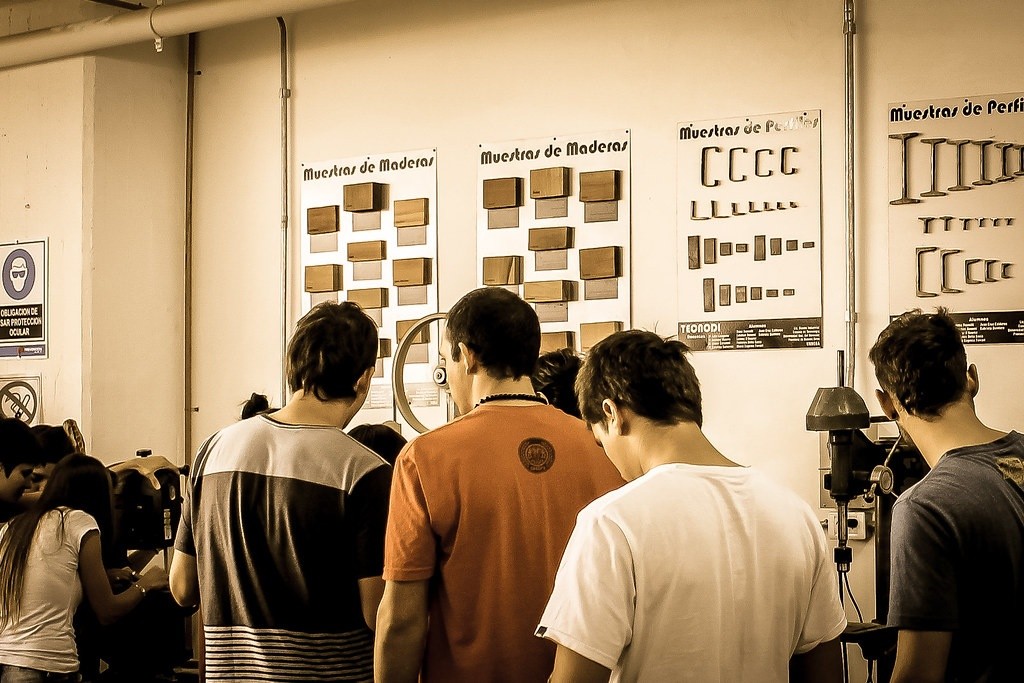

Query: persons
[868,306,1024,683]
[0,288,849,683]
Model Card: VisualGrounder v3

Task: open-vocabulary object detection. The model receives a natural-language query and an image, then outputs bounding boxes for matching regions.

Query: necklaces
[474,394,548,409]
[132,581,146,595]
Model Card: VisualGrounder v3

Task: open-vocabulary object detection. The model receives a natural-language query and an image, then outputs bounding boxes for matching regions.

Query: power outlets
[826,513,867,540]
